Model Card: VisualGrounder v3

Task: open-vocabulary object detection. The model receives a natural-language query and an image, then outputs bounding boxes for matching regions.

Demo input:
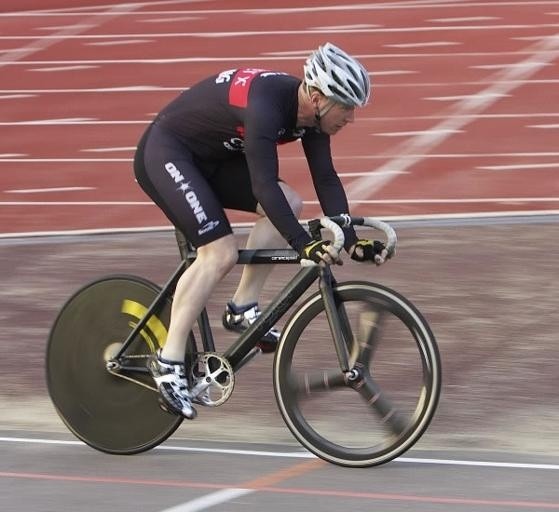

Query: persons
[135,43,396,420]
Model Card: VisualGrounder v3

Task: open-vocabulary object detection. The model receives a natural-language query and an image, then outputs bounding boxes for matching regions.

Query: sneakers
[147,348,197,419]
[223,303,281,343]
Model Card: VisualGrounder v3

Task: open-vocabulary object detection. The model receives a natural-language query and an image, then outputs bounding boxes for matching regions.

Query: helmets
[304,41,370,108]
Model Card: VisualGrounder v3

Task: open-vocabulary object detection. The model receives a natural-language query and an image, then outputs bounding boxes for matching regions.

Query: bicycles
[47,210,440,465]
